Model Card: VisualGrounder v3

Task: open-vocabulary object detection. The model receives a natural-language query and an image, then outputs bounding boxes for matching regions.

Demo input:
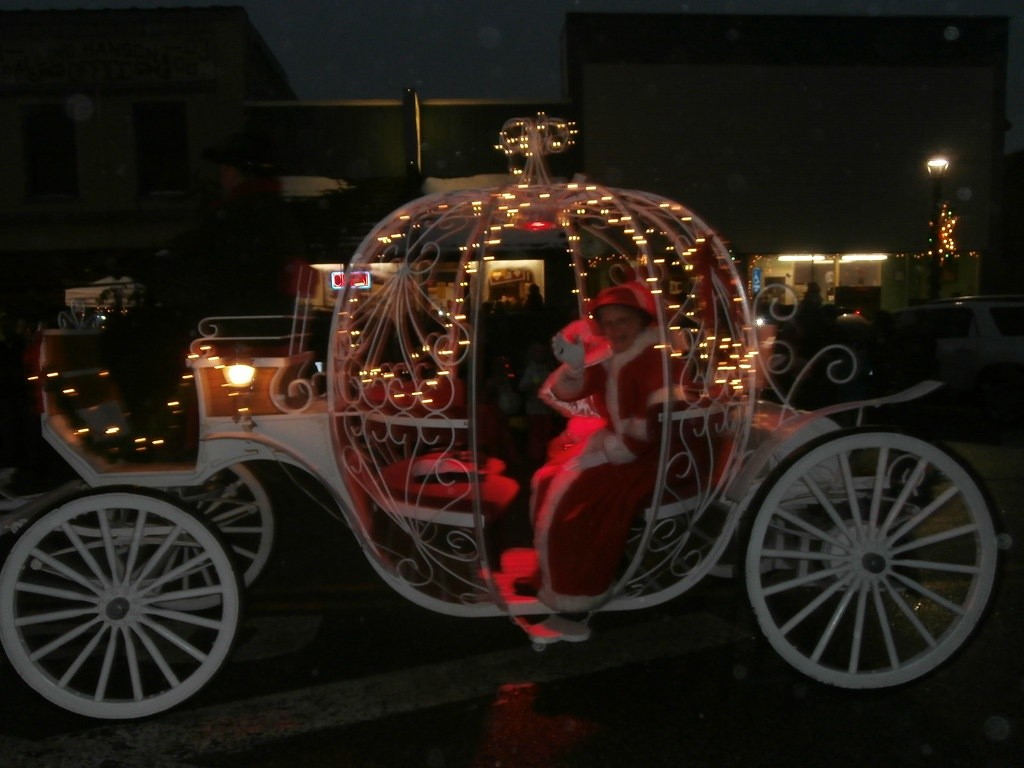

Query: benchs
[371,453,520,528]
[638,484,708,525]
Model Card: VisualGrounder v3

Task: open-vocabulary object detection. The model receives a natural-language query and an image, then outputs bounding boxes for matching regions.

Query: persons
[512,286,726,625]
[783,279,983,428]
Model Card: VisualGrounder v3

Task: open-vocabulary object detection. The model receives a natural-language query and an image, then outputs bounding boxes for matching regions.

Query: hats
[586,286,650,337]
[807,283,820,294]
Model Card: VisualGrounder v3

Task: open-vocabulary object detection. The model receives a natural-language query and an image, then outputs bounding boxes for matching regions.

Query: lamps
[222,346,257,424]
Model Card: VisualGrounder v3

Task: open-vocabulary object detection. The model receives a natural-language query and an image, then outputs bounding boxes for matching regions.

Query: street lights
[926,155,951,300]
